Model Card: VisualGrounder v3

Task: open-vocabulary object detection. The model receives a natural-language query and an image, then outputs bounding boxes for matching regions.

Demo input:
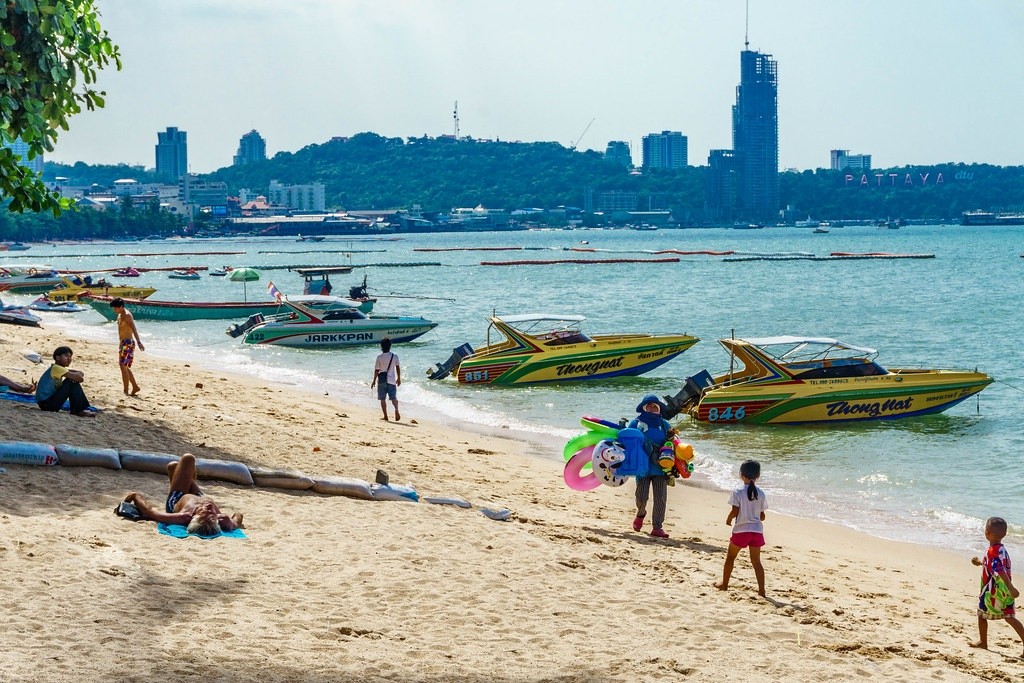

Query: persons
[110,297,145,395]
[0,375,37,394]
[36,346,96,416]
[713,459,768,597]
[187,268,195,273]
[971,517,1024,657]
[126,266,131,273]
[627,394,672,537]
[371,338,402,421]
[125,453,243,536]
[223,265,233,271]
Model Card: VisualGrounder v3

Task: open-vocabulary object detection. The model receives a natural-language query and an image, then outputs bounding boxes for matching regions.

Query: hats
[636,394,667,413]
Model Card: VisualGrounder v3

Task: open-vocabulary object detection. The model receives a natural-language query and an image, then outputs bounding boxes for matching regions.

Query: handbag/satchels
[378,372,387,383]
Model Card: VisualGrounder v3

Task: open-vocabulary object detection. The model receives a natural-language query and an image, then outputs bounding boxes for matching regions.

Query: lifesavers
[591,440,631,488]
[563,445,602,492]
[580,414,626,435]
[28,267,37,275]
[563,430,617,469]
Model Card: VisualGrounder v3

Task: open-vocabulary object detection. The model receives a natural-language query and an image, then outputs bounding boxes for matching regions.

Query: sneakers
[633,510,646,531]
[651,528,669,538]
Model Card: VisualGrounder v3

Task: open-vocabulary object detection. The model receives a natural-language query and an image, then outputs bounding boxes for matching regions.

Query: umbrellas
[225,268,262,305]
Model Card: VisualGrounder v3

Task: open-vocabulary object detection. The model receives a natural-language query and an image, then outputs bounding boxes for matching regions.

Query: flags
[268,283,281,299]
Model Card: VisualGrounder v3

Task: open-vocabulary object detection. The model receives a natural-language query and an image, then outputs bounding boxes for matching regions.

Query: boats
[957,209,1024,226]
[223,280,440,350]
[293,267,354,275]
[733,216,914,233]
[662,329,995,423]
[295,236,326,243]
[209,270,228,276]
[0,265,87,295]
[111,266,141,277]
[426,306,702,388]
[112,230,251,241]
[75,274,377,324]
[0,299,45,326]
[0,242,30,252]
[561,223,661,231]
[28,295,87,312]
[42,272,158,304]
[168,267,201,280]
